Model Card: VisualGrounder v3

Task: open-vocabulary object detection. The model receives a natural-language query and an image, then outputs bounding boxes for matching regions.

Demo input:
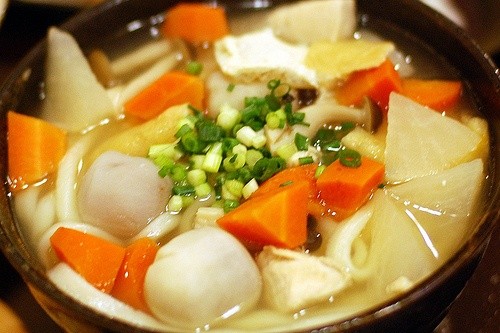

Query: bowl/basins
[1,0,499,333]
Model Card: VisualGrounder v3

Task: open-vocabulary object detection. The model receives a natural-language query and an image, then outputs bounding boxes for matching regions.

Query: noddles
[10,0,498,332]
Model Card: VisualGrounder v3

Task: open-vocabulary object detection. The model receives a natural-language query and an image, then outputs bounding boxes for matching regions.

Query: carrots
[5,1,464,310]
